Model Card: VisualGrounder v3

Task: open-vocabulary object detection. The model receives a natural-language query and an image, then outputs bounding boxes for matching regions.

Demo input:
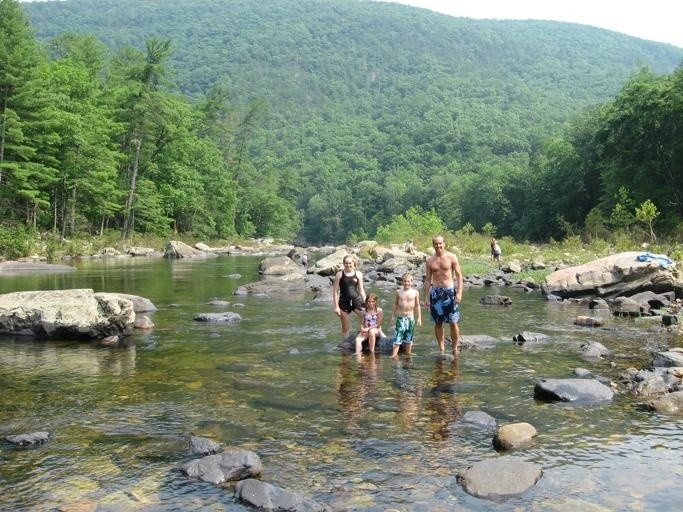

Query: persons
[424,356,464,445]
[423,235,462,354]
[332,254,366,336]
[336,354,357,415]
[492,241,500,262]
[387,358,422,430]
[490,238,494,261]
[389,273,422,356]
[354,293,383,356]
[358,363,378,404]
